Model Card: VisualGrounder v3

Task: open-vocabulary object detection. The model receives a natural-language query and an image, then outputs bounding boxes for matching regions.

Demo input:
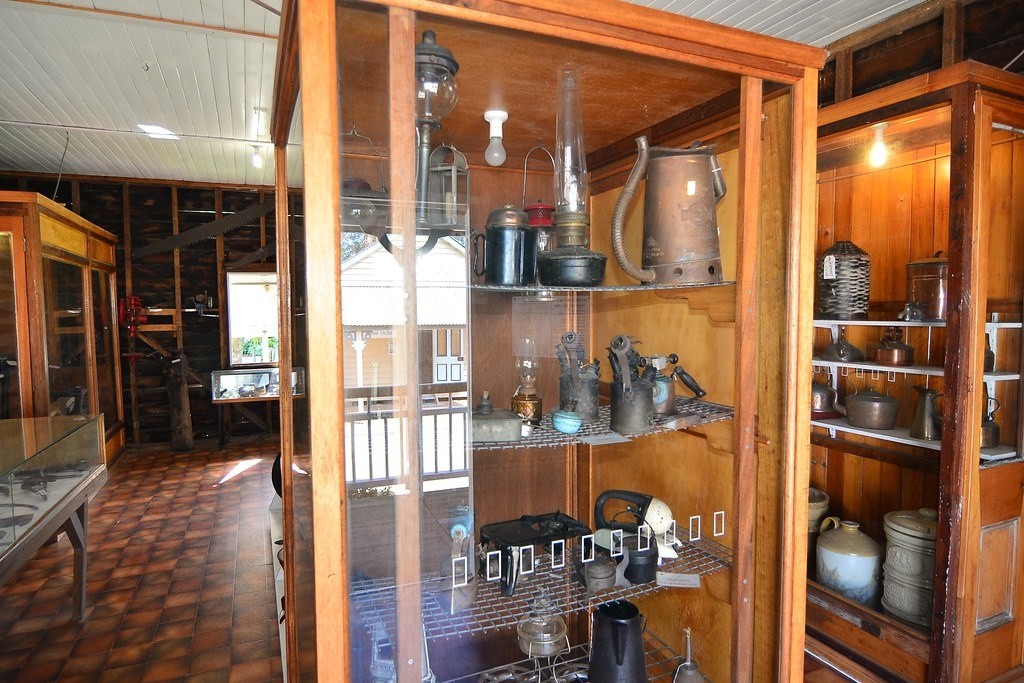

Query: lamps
[534,57,608,286]
[415,27,471,283]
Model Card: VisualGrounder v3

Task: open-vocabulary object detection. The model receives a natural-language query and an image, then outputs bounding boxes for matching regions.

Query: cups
[473,203,534,285]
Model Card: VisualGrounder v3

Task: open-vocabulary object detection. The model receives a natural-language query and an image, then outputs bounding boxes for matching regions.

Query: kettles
[828,369,899,430]
[873,328,914,366]
[820,327,865,363]
[812,369,832,412]
[610,511,658,584]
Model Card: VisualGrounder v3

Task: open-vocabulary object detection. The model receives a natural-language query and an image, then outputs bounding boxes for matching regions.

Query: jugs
[588,599,648,683]
[909,386,944,441]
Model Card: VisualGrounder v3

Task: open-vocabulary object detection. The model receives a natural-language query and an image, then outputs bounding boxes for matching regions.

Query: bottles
[817,522,880,608]
[817,240,870,322]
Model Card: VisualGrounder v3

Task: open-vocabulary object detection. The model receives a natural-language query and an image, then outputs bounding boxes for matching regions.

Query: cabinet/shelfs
[267,0,835,683]
[0,190,129,471]
[802,63,1024,683]
